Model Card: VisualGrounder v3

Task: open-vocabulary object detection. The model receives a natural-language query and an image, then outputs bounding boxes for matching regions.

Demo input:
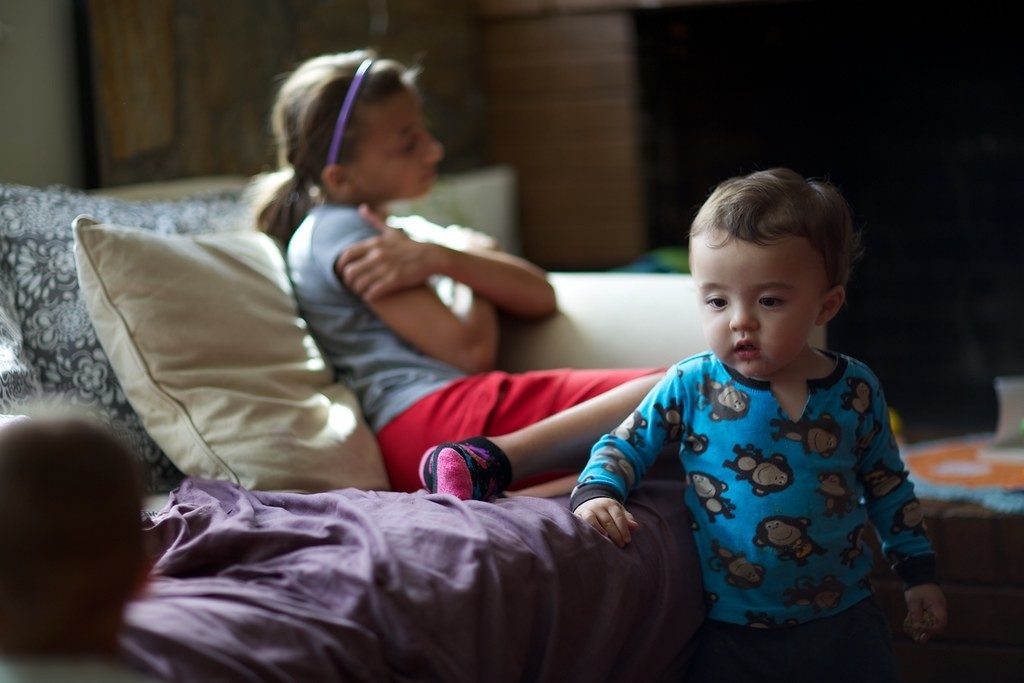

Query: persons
[568,168,946,683]
[0,415,161,683]
[257,50,671,501]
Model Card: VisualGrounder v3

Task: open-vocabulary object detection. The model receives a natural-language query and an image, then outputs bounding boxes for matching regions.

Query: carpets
[899,431,1024,516]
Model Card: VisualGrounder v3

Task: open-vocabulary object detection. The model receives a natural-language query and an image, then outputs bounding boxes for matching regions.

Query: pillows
[72,215,393,491]
[0,180,253,497]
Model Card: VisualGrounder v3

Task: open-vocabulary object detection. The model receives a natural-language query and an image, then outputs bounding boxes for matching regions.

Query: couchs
[0,164,827,683]
